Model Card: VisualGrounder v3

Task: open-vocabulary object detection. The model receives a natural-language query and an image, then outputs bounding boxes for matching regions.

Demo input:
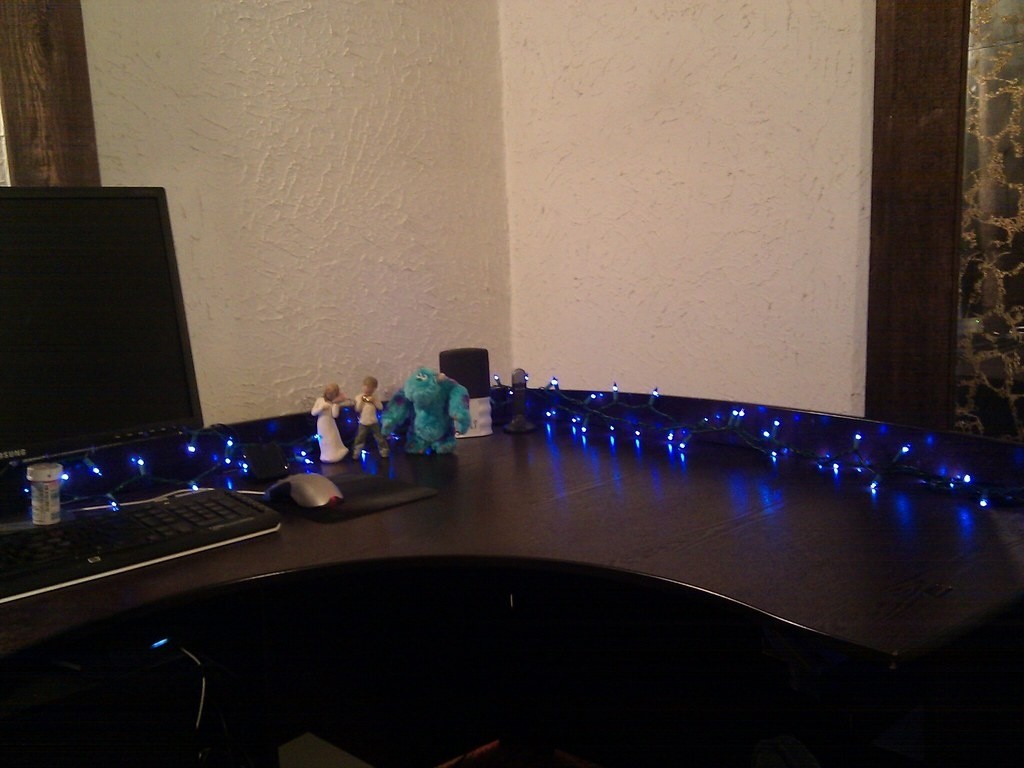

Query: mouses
[264,472,344,508]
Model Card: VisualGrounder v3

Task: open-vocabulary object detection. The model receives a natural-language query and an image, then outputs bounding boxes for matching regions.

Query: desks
[0,431,1024,675]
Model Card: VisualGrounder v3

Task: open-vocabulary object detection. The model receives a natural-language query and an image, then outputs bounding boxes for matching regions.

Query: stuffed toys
[380,360,470,454]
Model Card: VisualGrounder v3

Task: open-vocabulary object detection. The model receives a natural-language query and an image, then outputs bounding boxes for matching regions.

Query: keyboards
[0,487,283,604]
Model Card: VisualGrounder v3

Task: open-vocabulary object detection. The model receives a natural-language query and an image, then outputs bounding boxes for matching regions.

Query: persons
[354,377,390,459]
[311,382,349,463]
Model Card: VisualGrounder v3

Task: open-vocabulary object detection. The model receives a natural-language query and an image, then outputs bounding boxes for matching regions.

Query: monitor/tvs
[0,185,206,477]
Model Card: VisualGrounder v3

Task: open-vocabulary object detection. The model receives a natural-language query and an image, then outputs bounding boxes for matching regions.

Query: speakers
[439,347,494,438]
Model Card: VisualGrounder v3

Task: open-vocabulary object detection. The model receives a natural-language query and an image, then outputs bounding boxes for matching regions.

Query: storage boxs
[213,413,324,479]
